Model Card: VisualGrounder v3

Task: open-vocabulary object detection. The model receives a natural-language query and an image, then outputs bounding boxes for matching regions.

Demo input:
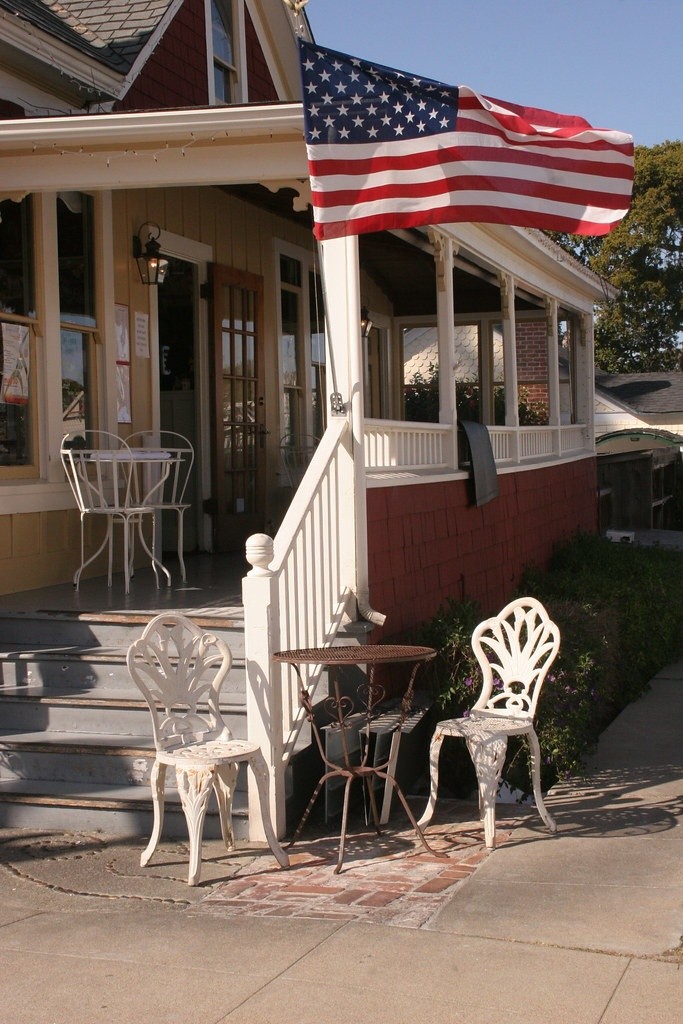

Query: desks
[69,456,185,590]
[271,644,450,876]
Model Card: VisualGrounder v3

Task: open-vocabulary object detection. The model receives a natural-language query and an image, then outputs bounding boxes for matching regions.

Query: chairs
[113,430,194,584]
[278,432,320,501]
[410,598,562,849]
[125,613,291,888]
[60,430,162,591]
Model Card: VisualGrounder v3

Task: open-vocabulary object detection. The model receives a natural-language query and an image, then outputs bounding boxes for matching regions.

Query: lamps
[133,220,169,286]
[361,306,373,337]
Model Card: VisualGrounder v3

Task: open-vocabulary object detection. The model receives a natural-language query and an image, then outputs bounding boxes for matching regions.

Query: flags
[296,37,635,236]
